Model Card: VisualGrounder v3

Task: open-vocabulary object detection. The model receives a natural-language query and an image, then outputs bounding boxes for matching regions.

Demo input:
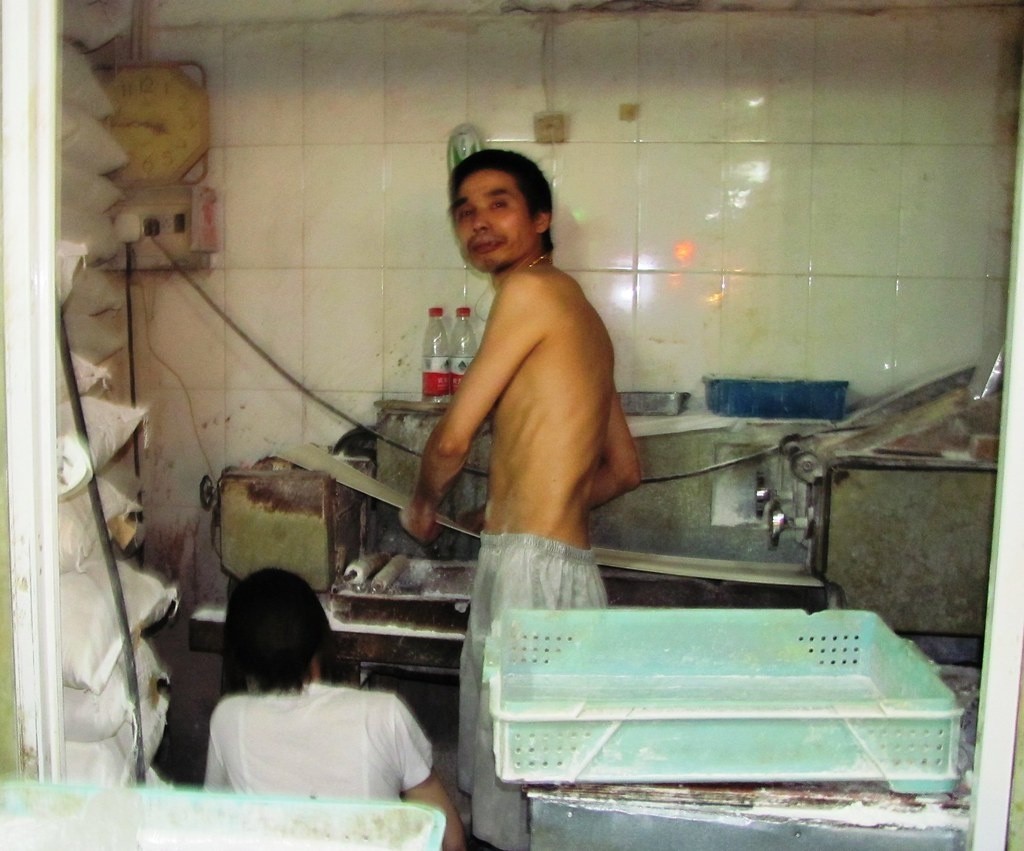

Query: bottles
[420,307,456,402]
[449,307,477,397]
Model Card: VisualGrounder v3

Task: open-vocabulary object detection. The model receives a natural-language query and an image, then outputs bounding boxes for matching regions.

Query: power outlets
[113,204,204,269]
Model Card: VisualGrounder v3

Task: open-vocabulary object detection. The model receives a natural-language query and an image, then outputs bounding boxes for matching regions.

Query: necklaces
[529,256,552,270]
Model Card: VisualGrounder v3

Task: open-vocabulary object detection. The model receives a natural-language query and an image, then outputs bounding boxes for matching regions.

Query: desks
[187,596,466,752]
[526,665,983,851]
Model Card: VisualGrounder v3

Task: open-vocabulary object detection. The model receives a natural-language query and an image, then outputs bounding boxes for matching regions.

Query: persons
[202,566,462,850]
[399,150,641,850]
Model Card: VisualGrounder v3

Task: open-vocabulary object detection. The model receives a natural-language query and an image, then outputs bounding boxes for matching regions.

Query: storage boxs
[483,609,962,795]
[703,375,851,418]
[0,783,446,851]
